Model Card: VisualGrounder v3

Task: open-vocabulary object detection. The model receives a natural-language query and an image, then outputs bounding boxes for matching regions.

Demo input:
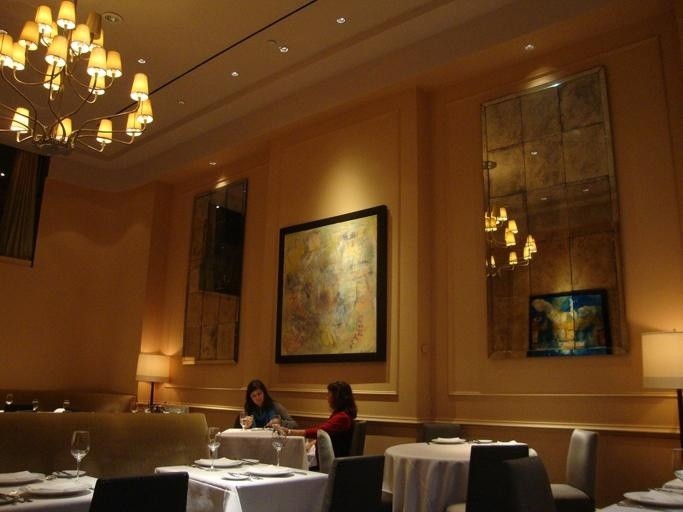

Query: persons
[271,380,359,472]
[241,379,298,431]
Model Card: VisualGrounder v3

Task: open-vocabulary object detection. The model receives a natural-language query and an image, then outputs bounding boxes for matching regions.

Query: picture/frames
[274,204,387,365]
[526,286,613,358]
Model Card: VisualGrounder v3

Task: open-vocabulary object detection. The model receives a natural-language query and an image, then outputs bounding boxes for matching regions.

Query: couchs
[0,389,210,477]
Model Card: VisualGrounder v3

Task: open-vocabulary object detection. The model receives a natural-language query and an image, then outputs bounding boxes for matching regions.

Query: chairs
[88,471,189,511]
[315,420,599,511]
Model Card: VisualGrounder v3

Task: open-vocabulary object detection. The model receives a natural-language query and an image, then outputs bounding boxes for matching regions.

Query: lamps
[485,206,538,279]
[0,0,154,155]
[638,328,683,448]
[135,353,170,407]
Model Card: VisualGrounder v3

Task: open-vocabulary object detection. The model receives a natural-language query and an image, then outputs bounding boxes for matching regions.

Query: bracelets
[287,428,292,435]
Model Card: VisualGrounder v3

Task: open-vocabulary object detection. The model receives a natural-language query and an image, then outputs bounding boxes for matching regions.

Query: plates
[223,473,249,481]
[0,472,44,487]
[53,468,86,479]
[24,481,92,497]
[193,458,243,468]
[245,467,297,476]
[430,438,466,444]
[620,490,682,508]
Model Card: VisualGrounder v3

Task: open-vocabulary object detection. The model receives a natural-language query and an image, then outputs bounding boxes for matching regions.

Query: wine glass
[128,400,189,414]
[270,427,287,468]
[238,411,248,433]
[4,393,71,412]
[69,430,92,484]
[204,427,222,473]
